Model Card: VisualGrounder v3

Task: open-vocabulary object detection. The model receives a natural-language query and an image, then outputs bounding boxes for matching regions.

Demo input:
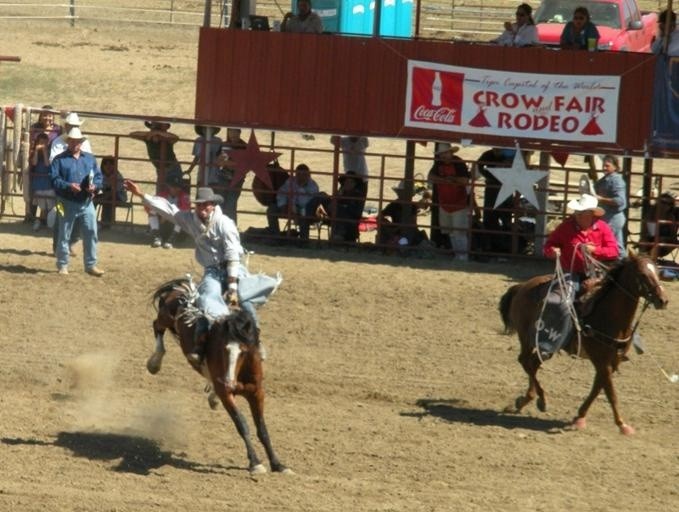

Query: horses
[497,249,670,436]
[144,277,299,477]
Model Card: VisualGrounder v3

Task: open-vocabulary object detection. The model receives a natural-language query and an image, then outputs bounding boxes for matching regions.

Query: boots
[151,228,164,247]
[163,228,178,248]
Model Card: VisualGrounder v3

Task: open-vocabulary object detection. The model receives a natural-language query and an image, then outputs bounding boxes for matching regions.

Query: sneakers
[86,265,106,276]
[186,351,202,368]
[263,227,280,234]
[58,268,69,275]
[256,340,267,361]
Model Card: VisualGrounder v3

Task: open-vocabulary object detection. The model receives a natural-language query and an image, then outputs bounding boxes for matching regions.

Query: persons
[589,153,629,260]
[122,178,267,367]
[280,0,325,35]
[497,3,540,49]
[557,6,601,49]
[649,9,679,58]
[540,193,644,360]
[637,193,679,257]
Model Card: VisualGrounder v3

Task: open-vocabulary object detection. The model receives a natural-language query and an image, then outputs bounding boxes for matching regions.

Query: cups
[647,223,656,237]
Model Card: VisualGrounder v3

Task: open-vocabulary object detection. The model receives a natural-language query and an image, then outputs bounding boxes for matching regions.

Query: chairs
[320,182,369,249]
[91,181,135,242]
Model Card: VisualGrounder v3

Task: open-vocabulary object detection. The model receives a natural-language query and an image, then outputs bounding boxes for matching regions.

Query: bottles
[88,166,94,186]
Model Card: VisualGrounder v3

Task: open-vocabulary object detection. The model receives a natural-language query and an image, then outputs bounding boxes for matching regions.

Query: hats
[63,127,87,143]
[567,193,606,217]
[194,125,221,136]
[392,180,416,196]
[157,165,183,187]
[62,112,86,126]
[143,119,170,131]
[189,186,225,205]
[433,142,459,155]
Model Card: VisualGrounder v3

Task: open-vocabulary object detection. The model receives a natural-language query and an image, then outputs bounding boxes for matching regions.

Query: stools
[281,216,322,246]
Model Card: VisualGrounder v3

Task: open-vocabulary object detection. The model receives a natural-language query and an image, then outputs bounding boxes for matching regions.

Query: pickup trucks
[533,0,663,54]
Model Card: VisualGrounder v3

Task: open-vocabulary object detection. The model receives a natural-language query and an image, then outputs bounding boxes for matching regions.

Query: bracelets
[228,281,238,292]
